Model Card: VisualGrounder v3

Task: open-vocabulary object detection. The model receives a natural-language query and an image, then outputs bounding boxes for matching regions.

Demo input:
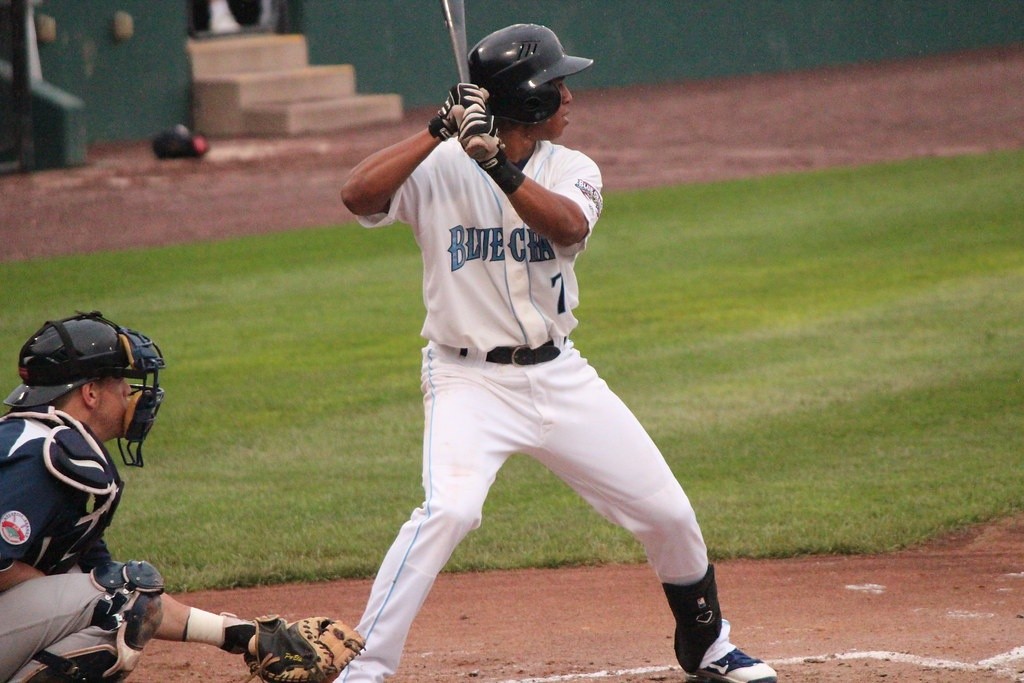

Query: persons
[0,312,366,683]
[331,23,779,683]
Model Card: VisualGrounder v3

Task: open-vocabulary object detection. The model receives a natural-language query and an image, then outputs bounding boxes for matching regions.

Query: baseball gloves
[242,614,366,683]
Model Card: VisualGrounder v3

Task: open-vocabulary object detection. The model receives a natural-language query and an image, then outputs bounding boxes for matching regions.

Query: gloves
[429,81,490,143]
[451,103,506,174]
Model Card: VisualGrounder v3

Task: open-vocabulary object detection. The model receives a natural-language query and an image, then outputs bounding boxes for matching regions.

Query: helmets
[3,313,130,406]
[468,23,592,124]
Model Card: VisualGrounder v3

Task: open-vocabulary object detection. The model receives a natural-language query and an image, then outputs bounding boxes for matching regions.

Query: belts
[459,337,567,368]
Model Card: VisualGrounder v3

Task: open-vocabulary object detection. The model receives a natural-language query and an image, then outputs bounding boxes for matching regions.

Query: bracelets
[182,607,224,646]
[489,159,527,194]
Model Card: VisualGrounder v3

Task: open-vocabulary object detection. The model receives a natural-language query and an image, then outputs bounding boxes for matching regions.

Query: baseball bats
[440,0,488,160]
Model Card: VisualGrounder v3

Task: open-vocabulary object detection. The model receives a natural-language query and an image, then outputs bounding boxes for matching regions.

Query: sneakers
[686,644,778,683]
